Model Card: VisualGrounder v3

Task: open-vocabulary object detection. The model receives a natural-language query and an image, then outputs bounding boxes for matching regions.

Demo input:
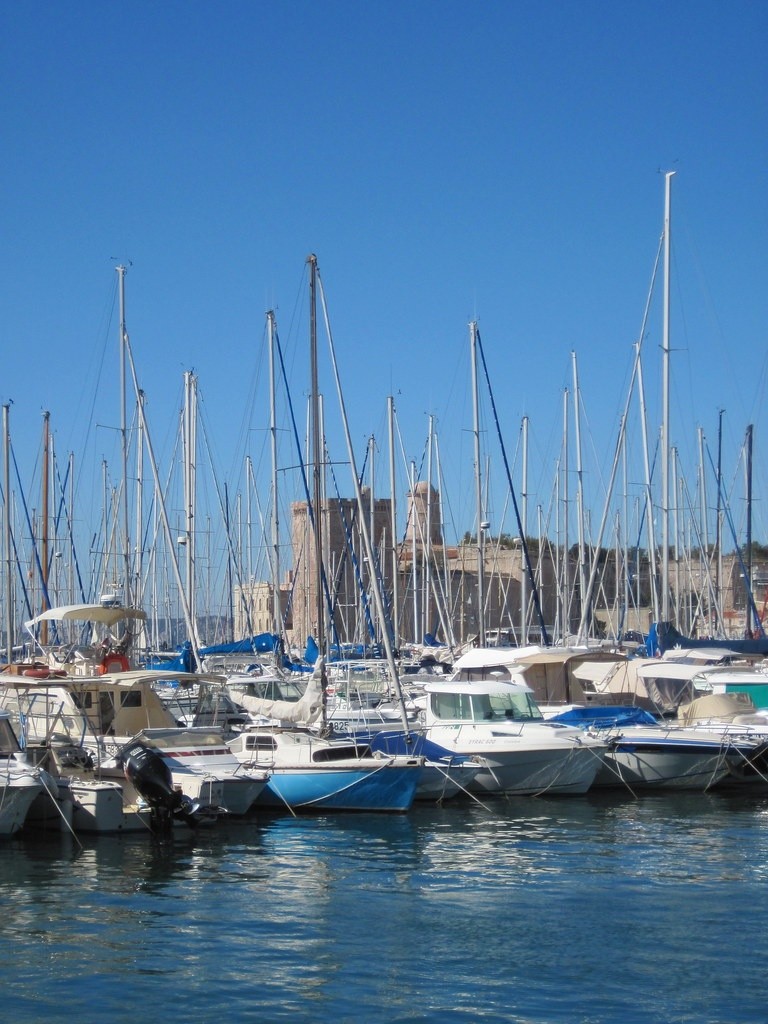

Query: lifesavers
[23,668,67,678]
[100,654,131,675]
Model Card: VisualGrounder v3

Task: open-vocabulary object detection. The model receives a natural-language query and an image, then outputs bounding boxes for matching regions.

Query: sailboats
[0,171,767,835]
